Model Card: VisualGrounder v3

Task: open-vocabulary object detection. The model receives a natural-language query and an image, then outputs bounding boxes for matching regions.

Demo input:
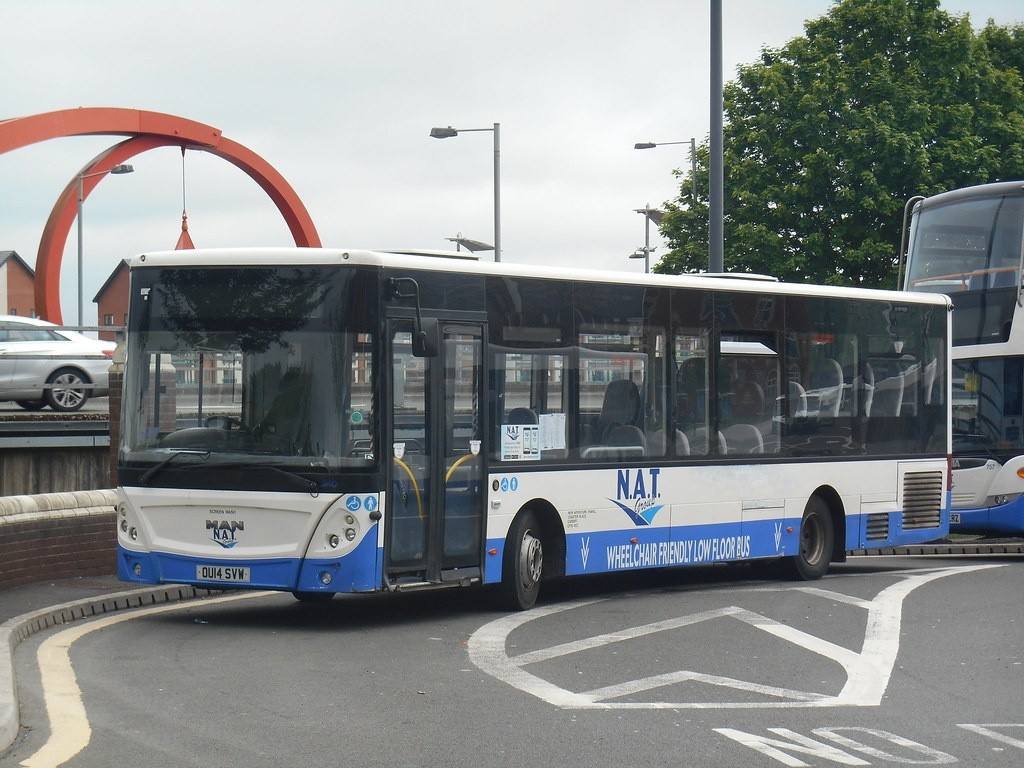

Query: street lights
[634,138,697,202]
[430,123,501,264]
[77,164,134,335]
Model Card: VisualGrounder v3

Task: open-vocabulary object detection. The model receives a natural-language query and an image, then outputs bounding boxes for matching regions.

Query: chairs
[606,425,648,456]
[788,381,808,417]
[649,427,691,456]
[842,359,875,417]
[686,427,727,454]
[807,358,844,417]
[679,356,705,371]
[596,379,641,442]
[507,407,539,424]
[642,357,676,383]
[873,359,905,417]
[736,380,766,424]
[725,424,764,454]
[251,371,312,443]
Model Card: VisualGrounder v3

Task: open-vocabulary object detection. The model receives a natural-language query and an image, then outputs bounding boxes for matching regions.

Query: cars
[0,314,118,412]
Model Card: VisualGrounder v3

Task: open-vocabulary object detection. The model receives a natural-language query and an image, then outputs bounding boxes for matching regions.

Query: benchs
[966,266,1020,290]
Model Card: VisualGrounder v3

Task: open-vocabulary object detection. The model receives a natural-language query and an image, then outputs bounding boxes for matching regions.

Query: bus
[897,181,1024,534]
[116,251,952,610]
